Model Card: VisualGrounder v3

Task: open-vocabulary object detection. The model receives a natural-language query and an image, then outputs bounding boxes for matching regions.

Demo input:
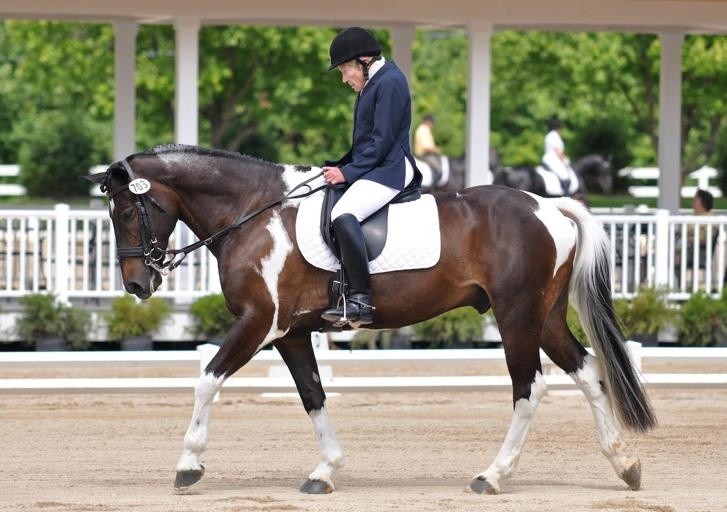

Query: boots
[321,213,374,324]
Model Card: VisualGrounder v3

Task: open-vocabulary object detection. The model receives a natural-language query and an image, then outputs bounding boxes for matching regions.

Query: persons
[675,188,719,266]
[319,25,423,325]
[414,113,444,175]
[541,116,571,194]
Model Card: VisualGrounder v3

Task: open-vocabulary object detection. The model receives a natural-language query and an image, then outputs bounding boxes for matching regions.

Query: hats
[328,26,382,70]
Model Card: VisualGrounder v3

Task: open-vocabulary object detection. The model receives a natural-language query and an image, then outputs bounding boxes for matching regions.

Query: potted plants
[347,306,487,350]
[613,285,727,347]
[15,293,234,349]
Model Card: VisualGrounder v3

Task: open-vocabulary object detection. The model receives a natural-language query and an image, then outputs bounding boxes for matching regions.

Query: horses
[78,141,660,496]
